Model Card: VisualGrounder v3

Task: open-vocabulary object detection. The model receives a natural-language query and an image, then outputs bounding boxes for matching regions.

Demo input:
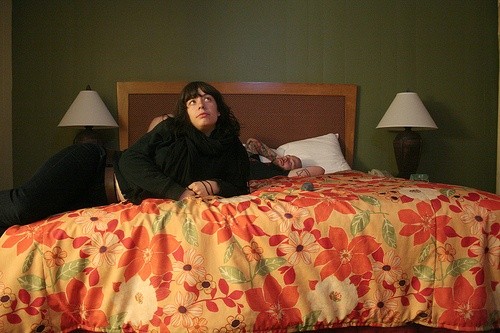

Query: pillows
[259,133,351,174]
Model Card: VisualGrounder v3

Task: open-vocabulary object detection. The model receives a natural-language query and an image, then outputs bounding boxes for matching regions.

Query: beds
[0,81,500,333]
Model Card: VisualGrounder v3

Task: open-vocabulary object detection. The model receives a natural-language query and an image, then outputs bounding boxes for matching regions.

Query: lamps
[57,84,118,146]
[376,92,438,179]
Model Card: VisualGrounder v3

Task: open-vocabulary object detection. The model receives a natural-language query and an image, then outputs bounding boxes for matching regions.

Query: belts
[105,150,117,205]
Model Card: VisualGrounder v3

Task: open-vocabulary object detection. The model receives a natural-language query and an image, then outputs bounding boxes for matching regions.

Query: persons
[0,81,250,231]
[146,113,325,180]
